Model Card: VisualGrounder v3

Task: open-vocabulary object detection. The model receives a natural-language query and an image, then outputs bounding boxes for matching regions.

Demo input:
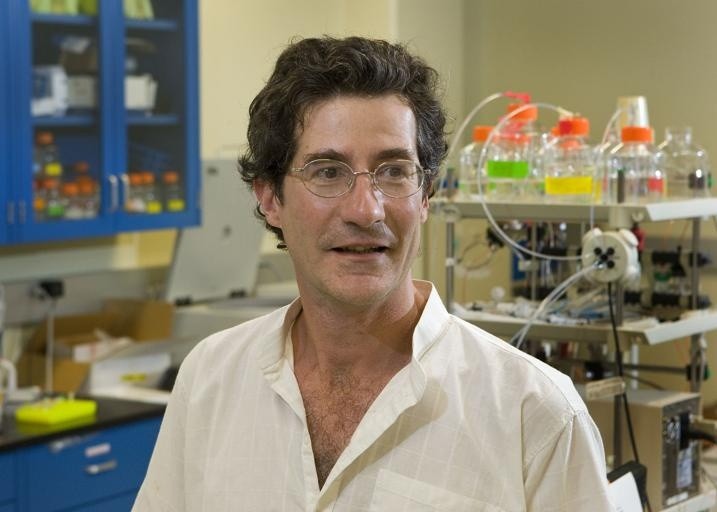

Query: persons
[127,36,617,510]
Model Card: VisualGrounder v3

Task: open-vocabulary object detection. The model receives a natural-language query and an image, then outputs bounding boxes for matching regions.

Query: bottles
[27,131,189,222]
[455,102,710,201]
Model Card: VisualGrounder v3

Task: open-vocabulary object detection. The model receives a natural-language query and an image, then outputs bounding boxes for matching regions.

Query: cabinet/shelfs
[423,186,717,346]
[0,391,167,512]
[0,0,204,247]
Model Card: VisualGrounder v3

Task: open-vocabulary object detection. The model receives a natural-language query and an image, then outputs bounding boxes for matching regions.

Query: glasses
[287,158,432,199]
